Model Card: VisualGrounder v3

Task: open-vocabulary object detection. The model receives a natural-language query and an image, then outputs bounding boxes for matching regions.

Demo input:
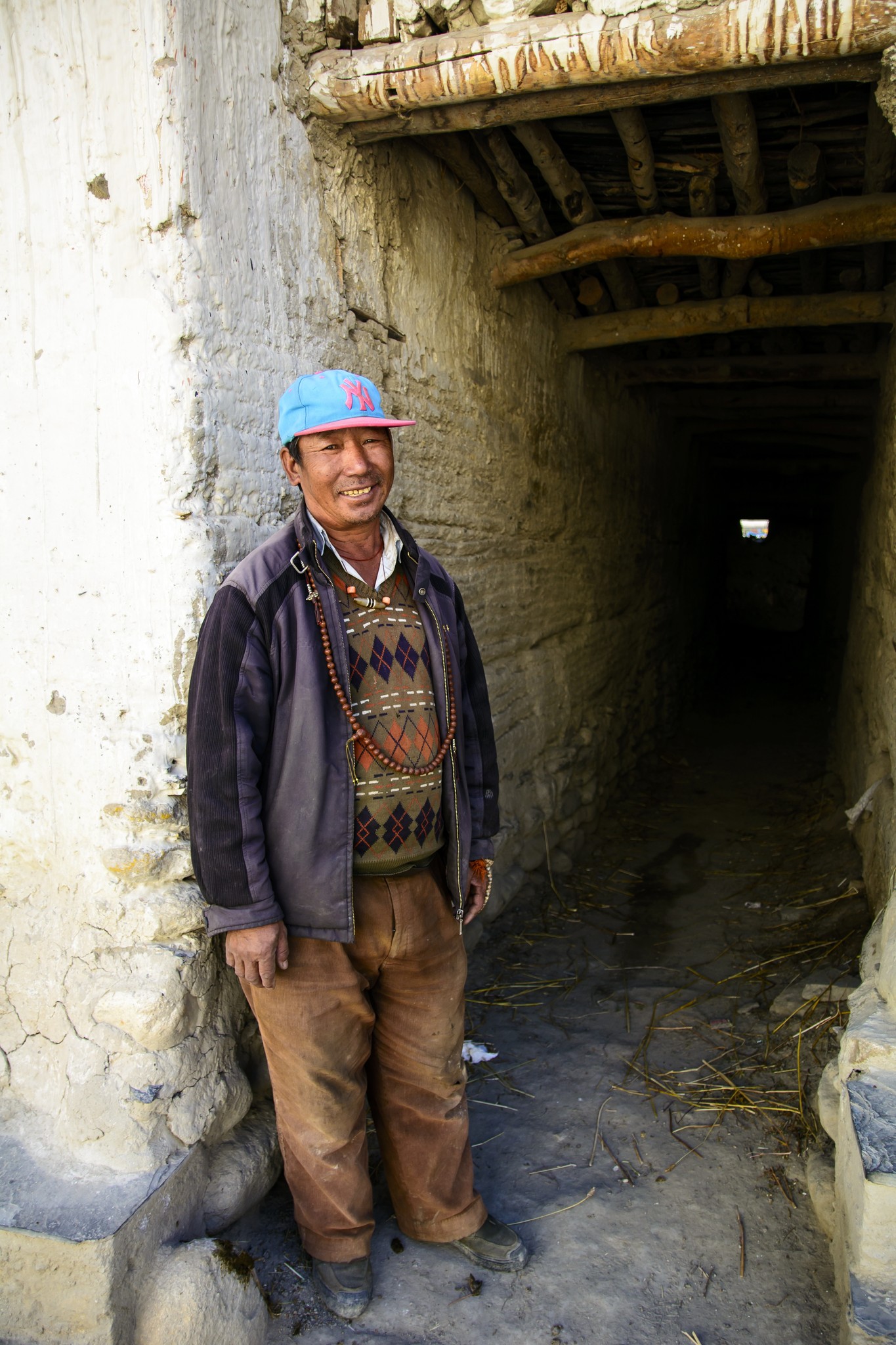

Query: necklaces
[329,572,402,613]
[335,547,381,562]
[293,534,459,777]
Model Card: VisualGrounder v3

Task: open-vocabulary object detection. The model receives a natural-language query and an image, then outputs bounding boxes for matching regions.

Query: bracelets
[469,858,494,914]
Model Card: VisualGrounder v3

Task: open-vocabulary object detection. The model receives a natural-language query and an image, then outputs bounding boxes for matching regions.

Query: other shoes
[446,1216,530,1271]
[312,1251,373,1319]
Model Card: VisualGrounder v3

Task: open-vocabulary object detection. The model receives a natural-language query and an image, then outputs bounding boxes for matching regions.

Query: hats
[277,369,416,446]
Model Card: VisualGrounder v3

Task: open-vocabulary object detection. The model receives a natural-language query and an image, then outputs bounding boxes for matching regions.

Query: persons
[185,370,530,1318]
